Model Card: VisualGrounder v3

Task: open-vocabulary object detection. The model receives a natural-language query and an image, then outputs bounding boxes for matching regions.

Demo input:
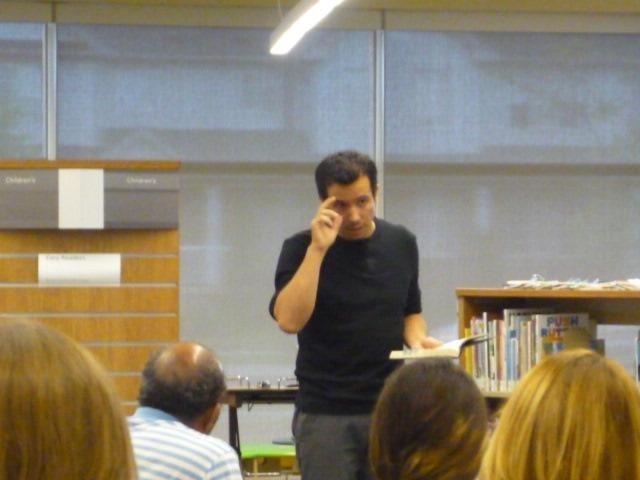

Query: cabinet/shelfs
[457,292,639,401]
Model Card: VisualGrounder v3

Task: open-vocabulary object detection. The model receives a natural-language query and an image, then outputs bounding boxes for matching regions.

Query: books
[462,306,604,391]
[389,332,490,359]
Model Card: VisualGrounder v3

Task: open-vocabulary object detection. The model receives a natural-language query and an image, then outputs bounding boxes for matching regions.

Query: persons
[266,149,444,480]
[124,342,243,480]
[367,358,489,479]
[0,315,139,479]
[474,347,640,479]
